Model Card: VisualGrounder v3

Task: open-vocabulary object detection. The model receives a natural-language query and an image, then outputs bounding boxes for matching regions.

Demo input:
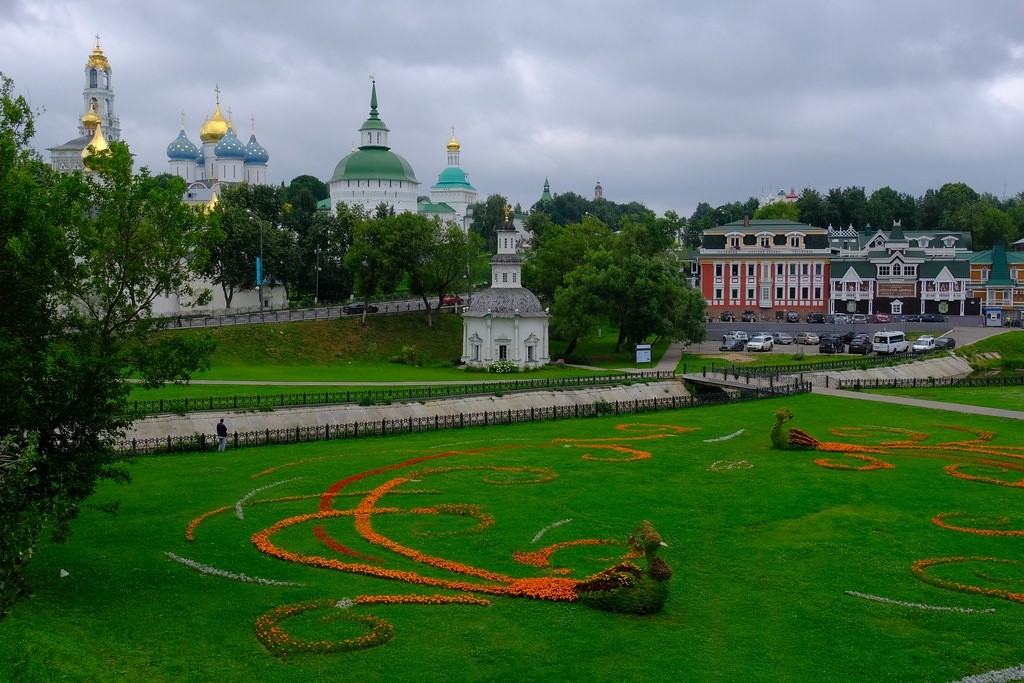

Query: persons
[979,313,1017,329]
[216,418,227,453]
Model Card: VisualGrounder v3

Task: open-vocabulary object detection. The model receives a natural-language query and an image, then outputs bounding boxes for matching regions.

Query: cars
[721,311,736,322]
[817,332,873,355]
[833,312,849,325]
[742,311,756,322]
[442,293,464,306]
[343,301,379,315]
[773,332,792,345]
[850,314,867,324]
[873,313,891,323]
[793,331,820,345]
[934,337,956,350]
[719,339,744,352]
[748,332,771,342]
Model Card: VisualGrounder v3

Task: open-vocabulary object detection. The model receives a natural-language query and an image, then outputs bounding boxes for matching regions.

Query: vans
[806,312,826,323]
[872,330,910,356]
[787,311,801,322]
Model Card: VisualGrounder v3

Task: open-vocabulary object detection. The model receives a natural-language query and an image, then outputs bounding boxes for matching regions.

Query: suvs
[912,337,935,354]
[747,336,774,352]
[722,330,749,344]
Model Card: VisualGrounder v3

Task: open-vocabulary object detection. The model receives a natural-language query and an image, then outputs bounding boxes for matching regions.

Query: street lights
[246,210,264,320]
